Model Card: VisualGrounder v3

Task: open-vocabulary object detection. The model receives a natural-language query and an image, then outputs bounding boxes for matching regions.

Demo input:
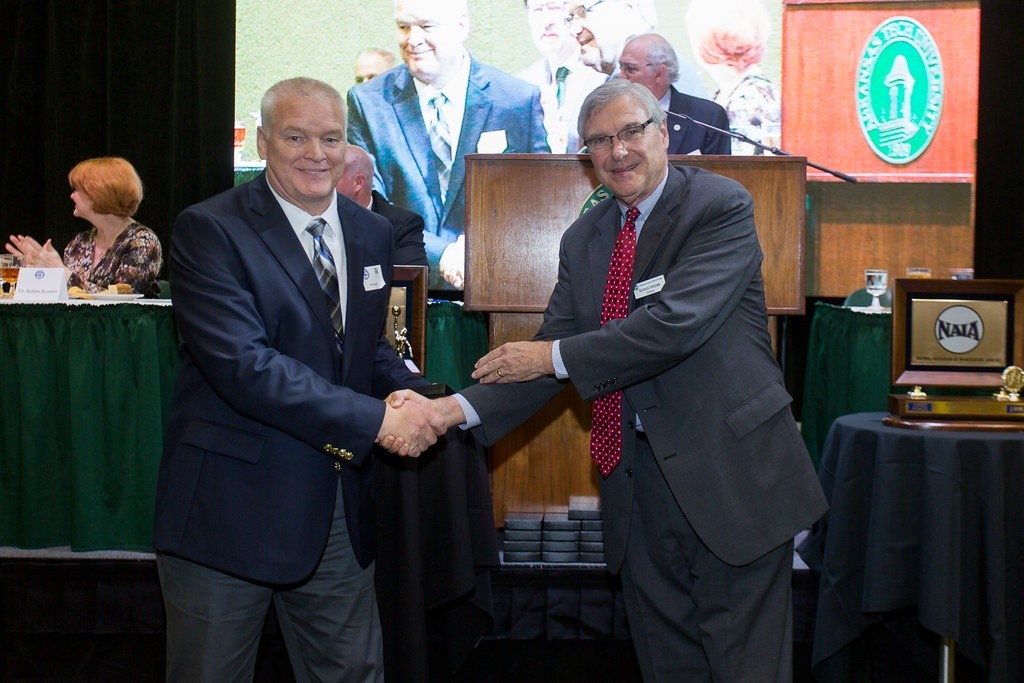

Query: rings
[497,369,504,377]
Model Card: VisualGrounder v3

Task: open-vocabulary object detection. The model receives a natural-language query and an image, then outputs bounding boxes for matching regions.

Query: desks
[0,291,491,562]
[794,411,1024,683]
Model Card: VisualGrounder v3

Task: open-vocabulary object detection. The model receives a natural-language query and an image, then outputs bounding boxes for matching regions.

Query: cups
[949,268,974,280]
[906,269,931,279]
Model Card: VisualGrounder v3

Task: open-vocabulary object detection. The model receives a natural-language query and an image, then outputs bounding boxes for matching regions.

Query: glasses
[562,1,606,24]
[619,63,654,74]
[583,118,653,155]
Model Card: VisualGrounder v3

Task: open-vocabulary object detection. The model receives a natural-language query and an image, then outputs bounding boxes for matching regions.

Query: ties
[590,208,641,480]
[304,217,343,368]
[555,66,569,112]
[428,95,453,208]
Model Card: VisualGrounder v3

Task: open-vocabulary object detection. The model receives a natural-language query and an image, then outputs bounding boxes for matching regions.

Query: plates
[87,293,144,301]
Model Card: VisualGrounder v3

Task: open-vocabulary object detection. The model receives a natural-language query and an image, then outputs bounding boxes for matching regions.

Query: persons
[382,78,830,683]
[5,157,162,299]
[618,33,730,156]
[348,0,554,292]
[151,77,449,682]
[337,145,429,267]
[528,0,660,153]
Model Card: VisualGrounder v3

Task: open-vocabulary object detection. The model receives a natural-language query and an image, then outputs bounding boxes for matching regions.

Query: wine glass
[0,254,26,299]
[865,270,888,312]
